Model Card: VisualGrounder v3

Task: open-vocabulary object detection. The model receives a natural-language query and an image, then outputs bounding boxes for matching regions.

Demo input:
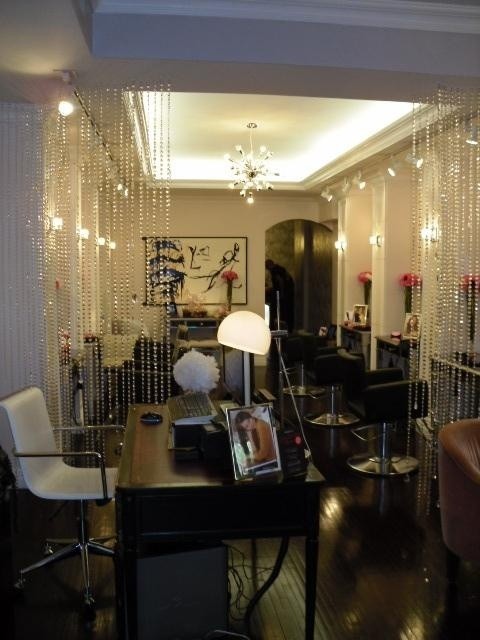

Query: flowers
[358,272,373,282]
[221,270,239,280]
[461,274,480,292]
[399,272,421,286]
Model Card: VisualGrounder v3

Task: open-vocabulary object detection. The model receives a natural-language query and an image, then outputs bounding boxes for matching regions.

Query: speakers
[199,431,231,470]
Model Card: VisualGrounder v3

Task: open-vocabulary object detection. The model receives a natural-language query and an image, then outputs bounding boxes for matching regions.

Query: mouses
[140,411,162,425]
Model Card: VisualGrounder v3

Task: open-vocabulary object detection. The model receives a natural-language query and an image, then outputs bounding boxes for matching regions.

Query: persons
[355,307,364,322]
[234,410,273,465]
[406,315,418,336]
[264,257,297,334]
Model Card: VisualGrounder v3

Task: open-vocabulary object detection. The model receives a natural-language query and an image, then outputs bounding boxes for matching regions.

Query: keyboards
[166,391,218,425]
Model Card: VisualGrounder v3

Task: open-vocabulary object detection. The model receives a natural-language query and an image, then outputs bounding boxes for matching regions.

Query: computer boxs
[129,539,228,640]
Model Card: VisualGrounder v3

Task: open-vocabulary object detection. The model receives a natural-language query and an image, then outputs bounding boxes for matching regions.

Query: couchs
[438,417,480,567]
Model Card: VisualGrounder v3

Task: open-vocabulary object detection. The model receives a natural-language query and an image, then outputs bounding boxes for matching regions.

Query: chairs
[297,328,365,426]
[2,385,123,609]
[274,318,331,396]
[335,347,429,477]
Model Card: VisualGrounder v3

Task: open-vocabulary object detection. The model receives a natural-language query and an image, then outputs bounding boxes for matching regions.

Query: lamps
[224,121,284,204]
[216,308,271,443]
[320,109,480,203]
[332,238,347,252]
[420,226,441,243]
[44,214,116,254]
[57,72,131,197]
[366,231,382,248]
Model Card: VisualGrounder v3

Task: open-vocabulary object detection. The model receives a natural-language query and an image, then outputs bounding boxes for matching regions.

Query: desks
[163,316,220,345]
[116,399,328,639]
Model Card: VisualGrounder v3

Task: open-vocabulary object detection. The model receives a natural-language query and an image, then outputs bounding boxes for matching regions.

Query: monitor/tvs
[218,316,255,418]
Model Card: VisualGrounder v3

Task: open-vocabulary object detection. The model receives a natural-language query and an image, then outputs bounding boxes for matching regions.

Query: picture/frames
[353,304,369,325]
[142,235,248,306]
[402,312,422,340]
[225,403,283,481]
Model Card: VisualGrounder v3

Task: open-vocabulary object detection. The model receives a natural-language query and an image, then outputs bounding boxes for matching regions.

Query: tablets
[170,425,207,448]
